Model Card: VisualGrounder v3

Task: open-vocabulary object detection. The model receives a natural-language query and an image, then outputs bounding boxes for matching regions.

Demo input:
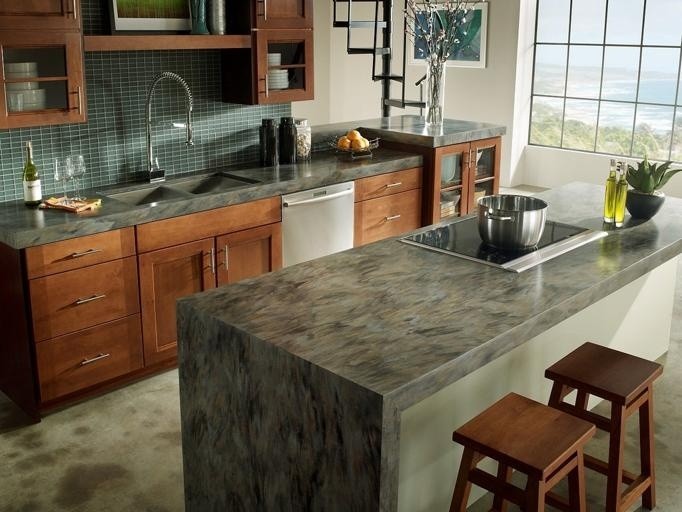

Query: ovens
[282,179,355,270]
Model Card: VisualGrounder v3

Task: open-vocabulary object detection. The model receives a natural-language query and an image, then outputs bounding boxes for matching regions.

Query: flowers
[400,1,478,124]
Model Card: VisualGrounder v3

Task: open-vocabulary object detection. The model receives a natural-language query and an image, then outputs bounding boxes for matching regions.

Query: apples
[338,129,369,150]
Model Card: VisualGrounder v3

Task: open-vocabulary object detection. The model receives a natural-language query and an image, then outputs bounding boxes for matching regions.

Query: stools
[452,391,596,512]
[545,343,664,512]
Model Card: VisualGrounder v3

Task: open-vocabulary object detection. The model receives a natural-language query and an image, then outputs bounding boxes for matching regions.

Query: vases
[425,55,446,127]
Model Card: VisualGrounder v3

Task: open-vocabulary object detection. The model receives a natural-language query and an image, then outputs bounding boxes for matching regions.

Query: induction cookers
[395,209,610,273]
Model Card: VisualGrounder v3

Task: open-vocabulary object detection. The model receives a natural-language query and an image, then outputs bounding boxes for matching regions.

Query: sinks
[95,183,186,206]
[165,172,262,195]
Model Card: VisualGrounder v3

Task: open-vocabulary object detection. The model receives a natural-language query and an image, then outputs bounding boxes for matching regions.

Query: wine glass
[188,0,226,35]
[52,154,86,206]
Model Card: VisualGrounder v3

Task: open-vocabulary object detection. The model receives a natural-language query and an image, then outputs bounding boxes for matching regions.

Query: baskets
[325,136,380,153]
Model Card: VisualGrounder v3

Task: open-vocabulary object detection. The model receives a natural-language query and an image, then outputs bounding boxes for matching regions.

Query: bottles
[278,118,297,163]
[596,223,625,272]
[22,143,42,208]
[258,119,277,166]
[295,117,311,162]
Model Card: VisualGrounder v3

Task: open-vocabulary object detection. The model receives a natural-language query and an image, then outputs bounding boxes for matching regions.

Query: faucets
[146,71,194,172]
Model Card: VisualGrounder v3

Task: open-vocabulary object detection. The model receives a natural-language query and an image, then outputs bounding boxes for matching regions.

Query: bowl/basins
[268,52,282,66]
[475,150,483,167]
[444,185,486,211]
[5,62,49,112]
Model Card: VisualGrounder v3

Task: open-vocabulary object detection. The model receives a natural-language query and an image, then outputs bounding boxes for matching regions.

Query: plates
[267,69,289,89]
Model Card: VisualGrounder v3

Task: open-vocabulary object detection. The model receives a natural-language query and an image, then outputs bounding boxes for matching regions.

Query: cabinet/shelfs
[0,227,146,423]
[133,195,281,375]
[354,168,422,246]
[422,136,502,228]
[221,1,314,105]
[1,0,88,129]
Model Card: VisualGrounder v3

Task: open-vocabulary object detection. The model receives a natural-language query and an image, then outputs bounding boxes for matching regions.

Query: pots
[473,244,544,299]
[477,196,548,250]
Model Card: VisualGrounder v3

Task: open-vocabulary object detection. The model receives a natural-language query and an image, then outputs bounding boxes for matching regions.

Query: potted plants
[616,154,682,219]
